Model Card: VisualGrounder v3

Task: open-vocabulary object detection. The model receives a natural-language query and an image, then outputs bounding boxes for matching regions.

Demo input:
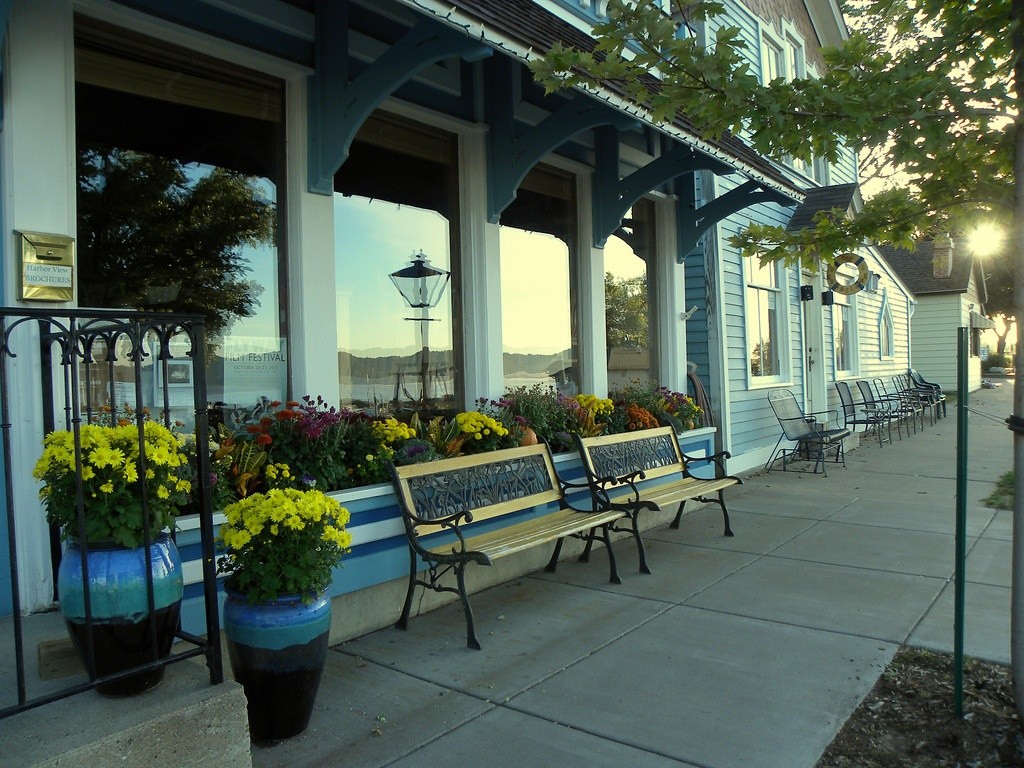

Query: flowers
[34,416,191,551]
[220,486,354,609]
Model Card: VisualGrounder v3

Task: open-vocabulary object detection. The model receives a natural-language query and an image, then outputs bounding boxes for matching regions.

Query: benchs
[569,418,743,578]
[392,434,632,651]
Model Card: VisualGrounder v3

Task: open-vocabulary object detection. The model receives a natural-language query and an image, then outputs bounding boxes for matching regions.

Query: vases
[57,528,185,699]
[221,587,333,745]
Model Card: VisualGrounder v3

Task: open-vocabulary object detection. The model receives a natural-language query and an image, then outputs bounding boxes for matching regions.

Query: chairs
[763,364,947,477]
